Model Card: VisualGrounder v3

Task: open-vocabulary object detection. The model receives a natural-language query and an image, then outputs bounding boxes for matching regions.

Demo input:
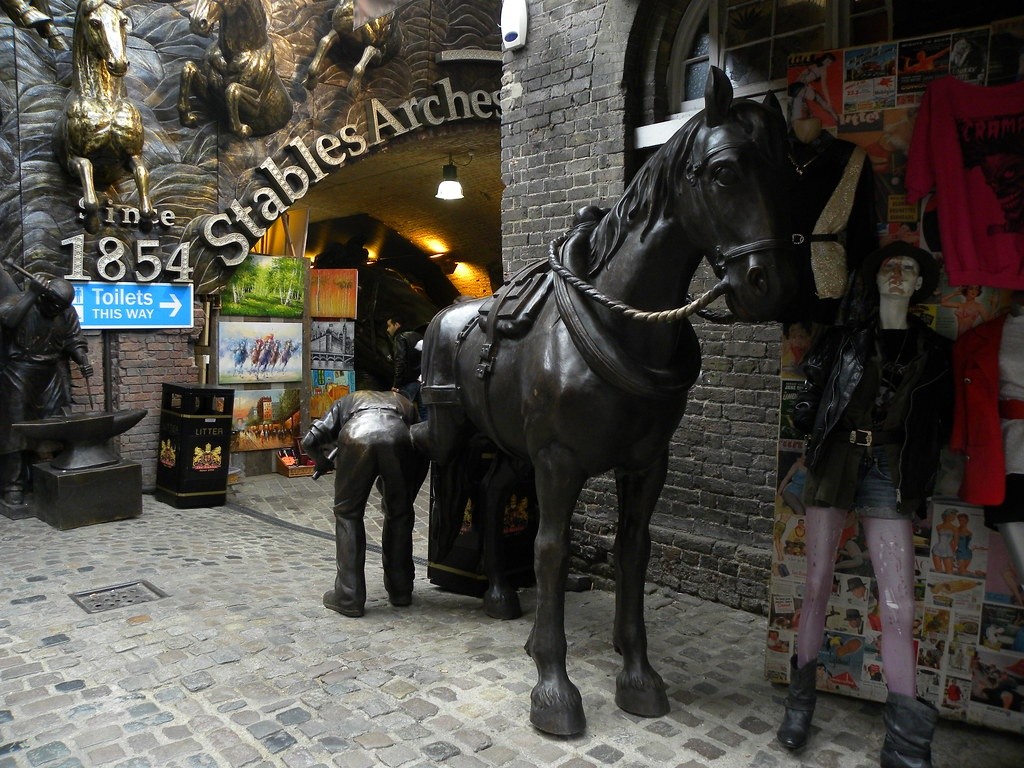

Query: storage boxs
[276,454,314,478]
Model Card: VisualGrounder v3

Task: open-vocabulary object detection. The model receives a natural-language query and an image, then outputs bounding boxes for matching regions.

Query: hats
[847,577,867,592]
[843,609,863,620]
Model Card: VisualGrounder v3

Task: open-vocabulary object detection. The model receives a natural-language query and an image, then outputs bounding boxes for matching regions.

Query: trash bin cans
[427,444,542,599]
[154,381,236,511]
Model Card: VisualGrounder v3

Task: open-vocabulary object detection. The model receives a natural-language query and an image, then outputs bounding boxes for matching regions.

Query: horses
[376,65,794,739]
[0,0,403,235]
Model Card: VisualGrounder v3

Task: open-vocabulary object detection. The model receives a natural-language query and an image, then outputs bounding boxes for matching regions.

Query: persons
[0,275,94,505]
[386,316,428,420]
[950,308,1024,581]
[300,390,419,618]
[790,239,956,768]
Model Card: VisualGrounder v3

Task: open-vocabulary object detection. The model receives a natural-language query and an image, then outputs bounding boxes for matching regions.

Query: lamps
[428,253,458,275]
[434,150,474,201]
